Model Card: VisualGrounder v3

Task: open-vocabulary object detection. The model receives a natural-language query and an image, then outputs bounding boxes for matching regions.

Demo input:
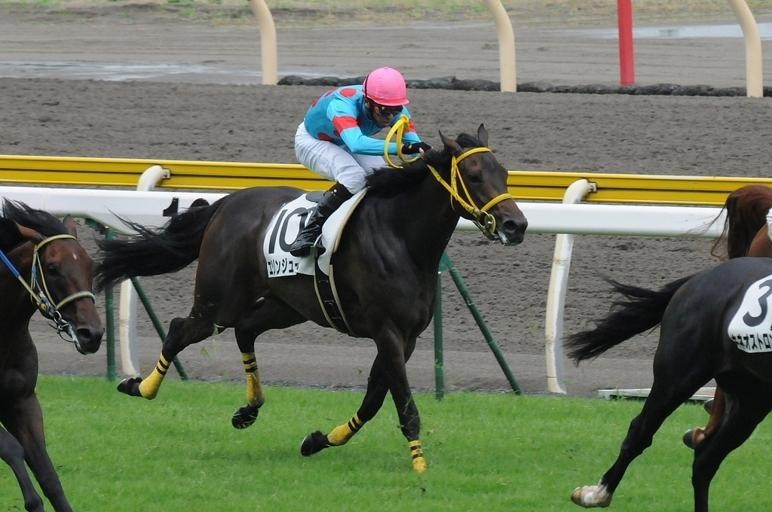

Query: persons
[287,66,432,259]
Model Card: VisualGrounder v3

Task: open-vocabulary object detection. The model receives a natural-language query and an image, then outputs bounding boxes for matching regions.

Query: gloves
[401,142,431,154]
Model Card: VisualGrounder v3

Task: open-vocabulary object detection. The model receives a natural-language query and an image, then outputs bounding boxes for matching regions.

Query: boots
[289,182,354,257]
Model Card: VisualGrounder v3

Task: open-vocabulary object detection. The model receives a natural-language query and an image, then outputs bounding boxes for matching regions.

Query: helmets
[362,67,410,107]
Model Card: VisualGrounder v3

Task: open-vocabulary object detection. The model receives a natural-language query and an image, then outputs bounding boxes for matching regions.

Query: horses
[0,197,105,512]
[94,122,528,475]
[560,257,769,510]
[681,181,770,449]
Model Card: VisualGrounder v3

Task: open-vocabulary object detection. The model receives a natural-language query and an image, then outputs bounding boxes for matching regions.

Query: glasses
[370,100,404,116]
